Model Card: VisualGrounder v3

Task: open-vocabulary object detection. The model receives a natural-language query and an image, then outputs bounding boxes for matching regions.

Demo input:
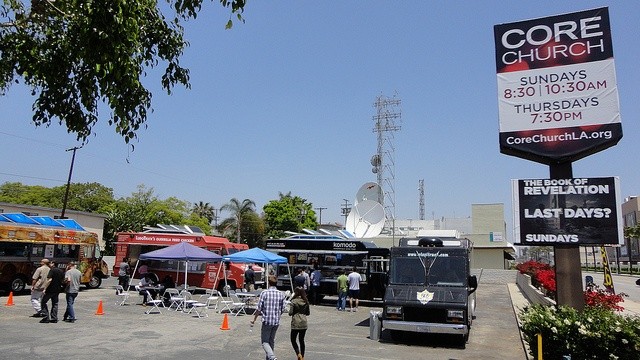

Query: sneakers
[49,319,57,323]
[40,319,48,323]
[66,319,74,323]
[34,313,43,317]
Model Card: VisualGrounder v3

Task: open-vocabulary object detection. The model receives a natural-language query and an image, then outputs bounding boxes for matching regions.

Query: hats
[302,266,307,270]
[65,262,78,271]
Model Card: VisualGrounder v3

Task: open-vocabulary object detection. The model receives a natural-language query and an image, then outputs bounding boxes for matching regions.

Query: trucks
[382,236,478,341]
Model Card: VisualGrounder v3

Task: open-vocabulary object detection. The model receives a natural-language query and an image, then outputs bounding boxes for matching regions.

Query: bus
[111,233,267,294]
[0,223,109,293]
[264,234,391,302]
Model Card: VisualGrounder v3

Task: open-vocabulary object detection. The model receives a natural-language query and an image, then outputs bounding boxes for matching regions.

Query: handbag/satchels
[119,271,126,276]
[60,278,70,292]
[34,279,46,291]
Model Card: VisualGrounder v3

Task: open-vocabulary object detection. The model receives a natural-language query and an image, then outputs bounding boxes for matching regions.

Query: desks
[206,289,218,310]
[136,285,164,304]
[176,286,196,294]
[231,293,260,309]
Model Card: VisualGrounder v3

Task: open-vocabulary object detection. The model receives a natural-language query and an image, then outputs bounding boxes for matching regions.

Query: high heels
[297,354,302,360]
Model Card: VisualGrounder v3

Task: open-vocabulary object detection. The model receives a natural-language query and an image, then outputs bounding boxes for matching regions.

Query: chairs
[229,293,247,317]
[182,291,198,312]
[242,289,250,302]
[282,293,297,312]
[191,294,211,318]
[168,289,184,312]
[229,290,235,293]
[144,290,162,315]
[282,290,291,303]
[114,285,130,306]
[235,288,248,304]
[218,291,232,315]
[161,288,177,305]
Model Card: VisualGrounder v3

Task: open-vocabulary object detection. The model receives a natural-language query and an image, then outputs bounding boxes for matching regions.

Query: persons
[62,260,82,321]
[40,260,63,322]
[310,265,323,305]
[300,266,310,292]
[335,269,350,311]
[250,275,286,360]
[286,287,310,360]
[115,256,174,306]
[29,259,51,316]
[245,265,256,291]
[293,267,304,287]
[348,266,363,312]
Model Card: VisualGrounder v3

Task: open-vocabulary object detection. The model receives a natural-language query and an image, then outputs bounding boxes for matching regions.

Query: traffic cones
[5,292,15,306]
[220,313,230,330]
[97,300,104,315]
